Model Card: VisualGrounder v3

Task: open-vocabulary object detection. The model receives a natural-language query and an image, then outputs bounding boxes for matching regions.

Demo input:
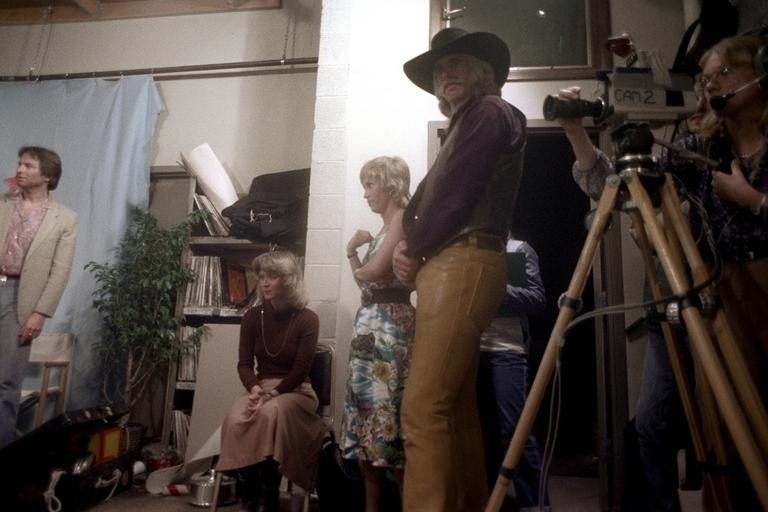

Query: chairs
[211,341,346,511]
[14,332,77,437]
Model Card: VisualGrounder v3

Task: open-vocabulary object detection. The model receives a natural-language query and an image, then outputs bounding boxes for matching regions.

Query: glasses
[695,63,754,87]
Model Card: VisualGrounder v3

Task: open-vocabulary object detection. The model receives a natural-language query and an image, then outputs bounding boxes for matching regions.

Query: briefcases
[0,402,136,512]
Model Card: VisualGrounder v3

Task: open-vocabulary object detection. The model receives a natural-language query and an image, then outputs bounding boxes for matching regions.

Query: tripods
[485,157,767,510]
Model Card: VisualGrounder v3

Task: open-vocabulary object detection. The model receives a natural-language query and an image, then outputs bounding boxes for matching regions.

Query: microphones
[710,77,761,111]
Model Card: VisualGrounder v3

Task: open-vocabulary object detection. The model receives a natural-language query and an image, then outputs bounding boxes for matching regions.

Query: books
[176,327,203,381]
[184,256,226,305]
[172,411,191,453]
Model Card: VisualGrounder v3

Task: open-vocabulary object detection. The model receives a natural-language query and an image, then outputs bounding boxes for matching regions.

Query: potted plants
[82,205,213,455]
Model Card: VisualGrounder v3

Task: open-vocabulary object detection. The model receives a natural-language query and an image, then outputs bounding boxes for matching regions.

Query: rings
[28,333,34,336]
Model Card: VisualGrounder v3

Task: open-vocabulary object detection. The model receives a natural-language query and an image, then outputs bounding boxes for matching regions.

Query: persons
[686,73,715,131]
[340,155,414,511]
[214,249,318,512]
[475,227,545,508]
[556,29,767,510]
[1,145,79,453]
[392,26,528,512]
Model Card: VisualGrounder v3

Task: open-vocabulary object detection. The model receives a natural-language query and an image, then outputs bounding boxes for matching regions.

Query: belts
[0,275,19,283]
[446,236,502,252]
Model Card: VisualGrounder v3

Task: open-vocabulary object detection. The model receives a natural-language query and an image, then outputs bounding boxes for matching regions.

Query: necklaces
[261,309,295,356]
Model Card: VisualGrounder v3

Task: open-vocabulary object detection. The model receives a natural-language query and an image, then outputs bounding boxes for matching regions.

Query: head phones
[739,26,767,83]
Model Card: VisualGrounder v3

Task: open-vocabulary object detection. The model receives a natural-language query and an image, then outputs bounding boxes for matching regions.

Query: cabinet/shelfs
[162,237,306,447]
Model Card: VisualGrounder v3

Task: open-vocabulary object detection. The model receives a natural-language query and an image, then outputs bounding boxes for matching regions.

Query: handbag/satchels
[189,468,237,507]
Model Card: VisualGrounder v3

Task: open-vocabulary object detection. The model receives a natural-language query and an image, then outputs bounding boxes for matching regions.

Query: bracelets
[754,196,766,217]
[343,250,360,261]
[266,392,274,398]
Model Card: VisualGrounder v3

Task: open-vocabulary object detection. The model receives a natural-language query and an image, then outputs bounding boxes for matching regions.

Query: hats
[403,28,510,94]
